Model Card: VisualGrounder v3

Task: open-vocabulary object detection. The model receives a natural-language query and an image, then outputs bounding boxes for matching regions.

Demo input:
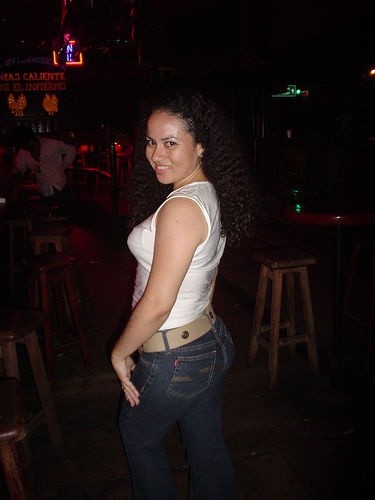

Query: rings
[121,384,125,391]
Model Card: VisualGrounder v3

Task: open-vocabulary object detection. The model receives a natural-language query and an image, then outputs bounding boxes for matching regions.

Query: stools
[6,177,68,255]
[1,378,39,500]
[20,251,94,386]
[0,306,63,456]
[249,246,324,391]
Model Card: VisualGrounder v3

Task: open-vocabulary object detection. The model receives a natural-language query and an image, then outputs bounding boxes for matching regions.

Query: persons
[7,125,78,226]
[110,87,260,500]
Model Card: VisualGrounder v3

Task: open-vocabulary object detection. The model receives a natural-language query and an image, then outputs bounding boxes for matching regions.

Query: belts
[139,302,217,353]
